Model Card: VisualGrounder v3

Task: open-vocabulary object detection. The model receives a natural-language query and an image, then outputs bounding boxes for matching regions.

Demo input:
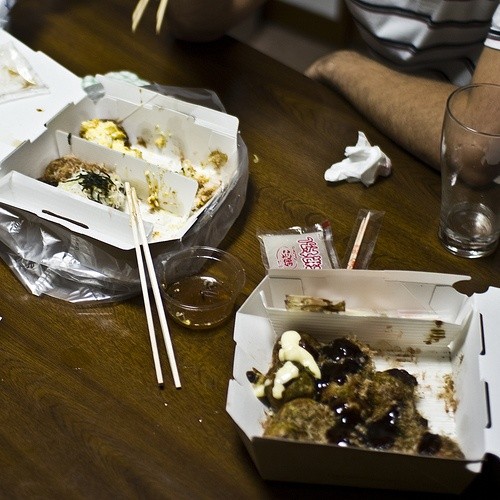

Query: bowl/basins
[158,245,246,330]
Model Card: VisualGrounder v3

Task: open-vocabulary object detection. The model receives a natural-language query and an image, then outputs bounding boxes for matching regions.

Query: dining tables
[0,0,500,500]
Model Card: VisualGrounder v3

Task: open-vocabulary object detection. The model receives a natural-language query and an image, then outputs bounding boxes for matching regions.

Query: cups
[437,83,500,259]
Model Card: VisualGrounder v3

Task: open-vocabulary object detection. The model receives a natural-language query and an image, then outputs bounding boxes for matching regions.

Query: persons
[165,0,500,186]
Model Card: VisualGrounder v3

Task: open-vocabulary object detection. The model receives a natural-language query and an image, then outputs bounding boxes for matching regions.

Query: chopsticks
[125,181,182,388]
[346,211,372,269]
[131,0,169,34]
